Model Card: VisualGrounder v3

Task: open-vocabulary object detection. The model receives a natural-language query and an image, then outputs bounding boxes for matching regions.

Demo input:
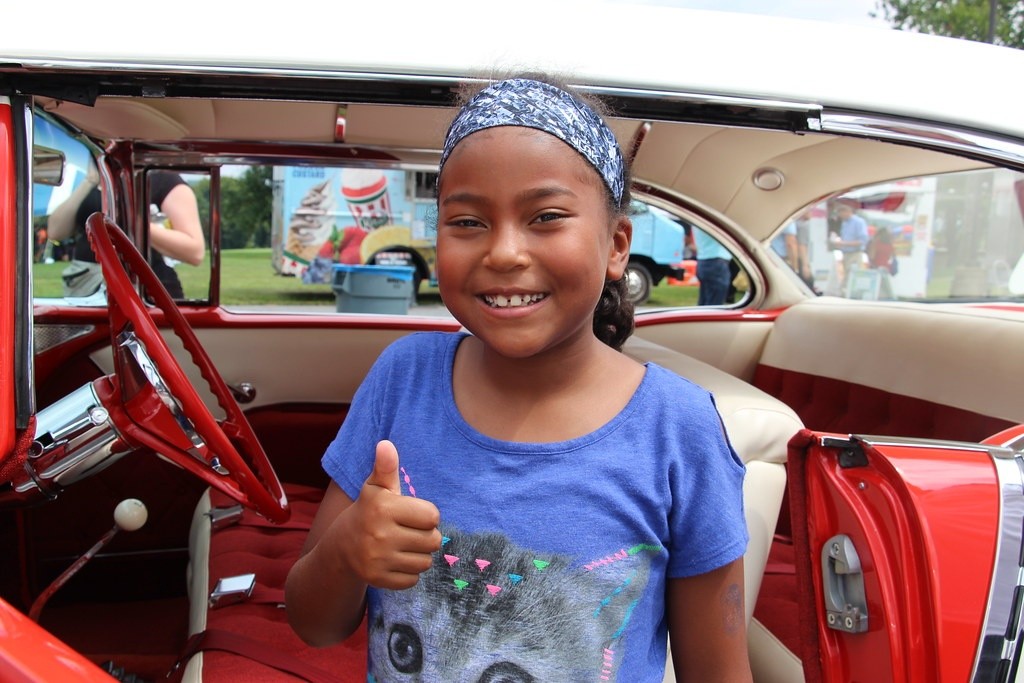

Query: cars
[1,0,1021,683]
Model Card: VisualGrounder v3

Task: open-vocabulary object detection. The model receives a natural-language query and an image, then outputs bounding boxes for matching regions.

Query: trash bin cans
[329,262,416,314]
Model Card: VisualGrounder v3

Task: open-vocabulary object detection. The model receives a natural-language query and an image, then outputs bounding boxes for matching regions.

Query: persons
[867,227,897,300]
[34,223,73,262]
[691,224,740,306]
[833,205,867,299]
[286,71,753,683]
[771,211,823,298]
[47,154,204,301]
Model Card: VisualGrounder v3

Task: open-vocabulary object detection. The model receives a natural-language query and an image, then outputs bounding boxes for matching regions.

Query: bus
[267,166,683,311]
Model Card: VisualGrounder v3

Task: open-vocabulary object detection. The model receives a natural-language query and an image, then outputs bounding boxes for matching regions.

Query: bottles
[149,203,181,267]
